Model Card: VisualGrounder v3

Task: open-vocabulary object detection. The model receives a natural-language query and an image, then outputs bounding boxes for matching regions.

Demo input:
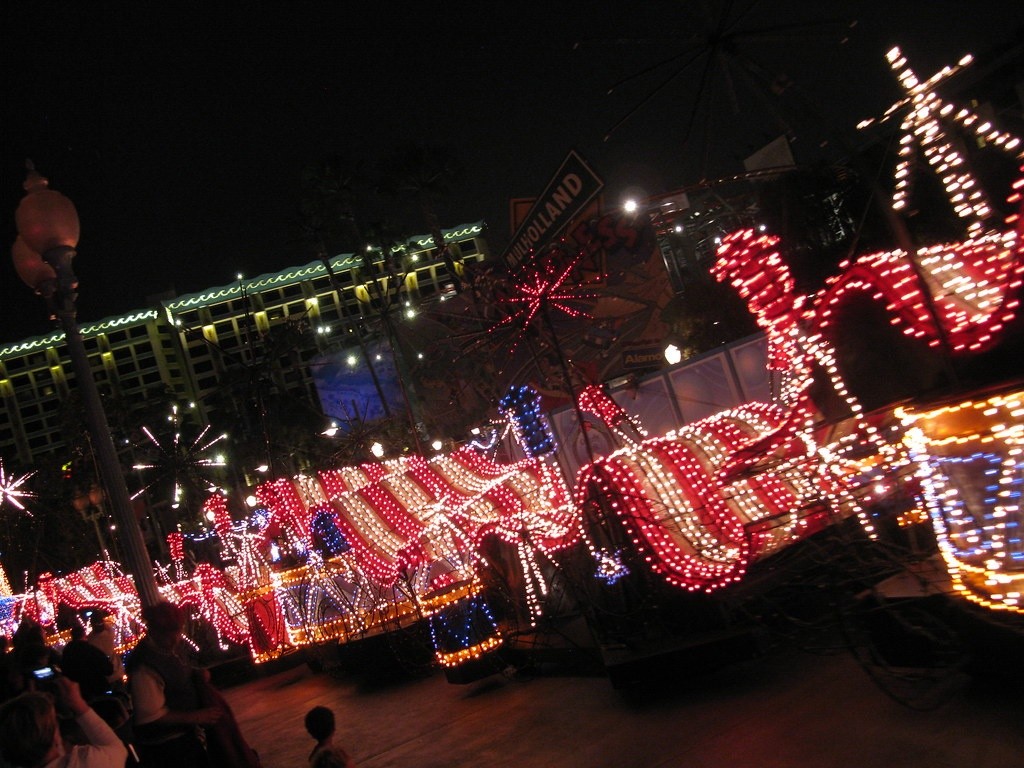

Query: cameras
[85,611,94,617]
[32,665,58,680]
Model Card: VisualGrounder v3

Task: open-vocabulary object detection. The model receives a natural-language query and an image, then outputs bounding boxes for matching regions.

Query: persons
[304,705,355,768]
[0,599,224,768]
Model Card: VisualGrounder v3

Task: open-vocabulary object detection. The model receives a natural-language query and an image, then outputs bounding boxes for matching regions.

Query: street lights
[11,158,159,618]
